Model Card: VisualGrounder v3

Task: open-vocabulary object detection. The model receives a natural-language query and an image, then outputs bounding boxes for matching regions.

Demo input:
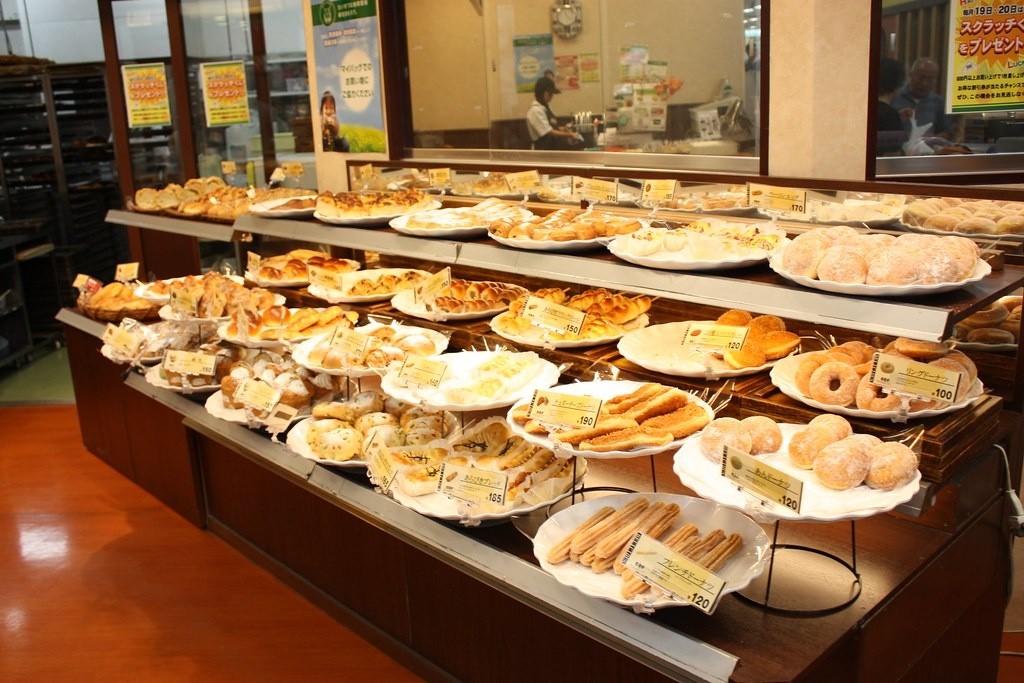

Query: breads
[85,251,921,604]
[135,170,1024,290]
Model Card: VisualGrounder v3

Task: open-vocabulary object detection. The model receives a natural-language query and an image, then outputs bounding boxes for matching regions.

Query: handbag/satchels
[901,110,935,157]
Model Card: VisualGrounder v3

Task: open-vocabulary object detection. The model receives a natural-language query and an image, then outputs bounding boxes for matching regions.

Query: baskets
[76,297,164,323]
[127,199,234,227]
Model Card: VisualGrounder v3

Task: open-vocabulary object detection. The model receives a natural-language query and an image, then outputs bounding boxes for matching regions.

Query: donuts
[949,295,1024,347]
[794,338,978,411]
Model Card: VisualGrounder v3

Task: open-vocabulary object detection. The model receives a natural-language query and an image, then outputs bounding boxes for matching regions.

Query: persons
[526,77,579,150]
[320,91,350,153]
[744,43,761,71]
[875,57,959,156]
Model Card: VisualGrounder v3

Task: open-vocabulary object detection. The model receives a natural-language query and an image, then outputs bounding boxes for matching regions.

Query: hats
[536,76,562,93]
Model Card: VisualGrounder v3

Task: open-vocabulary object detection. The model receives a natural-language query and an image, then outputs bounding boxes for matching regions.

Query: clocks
[550,0,583,40]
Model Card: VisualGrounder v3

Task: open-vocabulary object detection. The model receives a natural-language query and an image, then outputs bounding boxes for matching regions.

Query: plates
[102,194,788,520]
[673,422,922,520]
[768,256,991,295]
[947,296,1024,351]
[534,493,771,608]
[389,176,1024,238]
[770,348,982,419]
